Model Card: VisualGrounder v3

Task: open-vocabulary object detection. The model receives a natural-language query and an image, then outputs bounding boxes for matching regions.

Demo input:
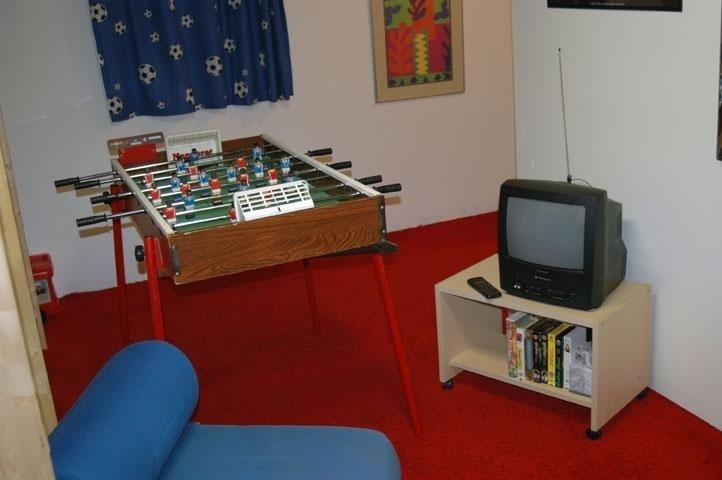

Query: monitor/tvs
[496,179,627,311]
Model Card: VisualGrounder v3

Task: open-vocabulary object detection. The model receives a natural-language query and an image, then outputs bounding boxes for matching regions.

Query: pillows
[47,341,199,480]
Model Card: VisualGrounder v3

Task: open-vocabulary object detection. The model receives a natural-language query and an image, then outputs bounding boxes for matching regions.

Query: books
[505,311,593,401]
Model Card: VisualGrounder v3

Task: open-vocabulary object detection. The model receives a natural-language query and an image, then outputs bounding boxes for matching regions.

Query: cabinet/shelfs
[434,254,651,439]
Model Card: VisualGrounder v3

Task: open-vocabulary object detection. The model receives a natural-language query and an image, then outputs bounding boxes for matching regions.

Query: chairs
[48,341,401,480]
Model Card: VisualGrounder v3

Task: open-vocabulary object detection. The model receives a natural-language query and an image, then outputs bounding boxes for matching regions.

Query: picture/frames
[371,0,465,103]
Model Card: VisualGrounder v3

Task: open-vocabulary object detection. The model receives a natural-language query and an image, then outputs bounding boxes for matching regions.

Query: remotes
[467,276,501,299]
